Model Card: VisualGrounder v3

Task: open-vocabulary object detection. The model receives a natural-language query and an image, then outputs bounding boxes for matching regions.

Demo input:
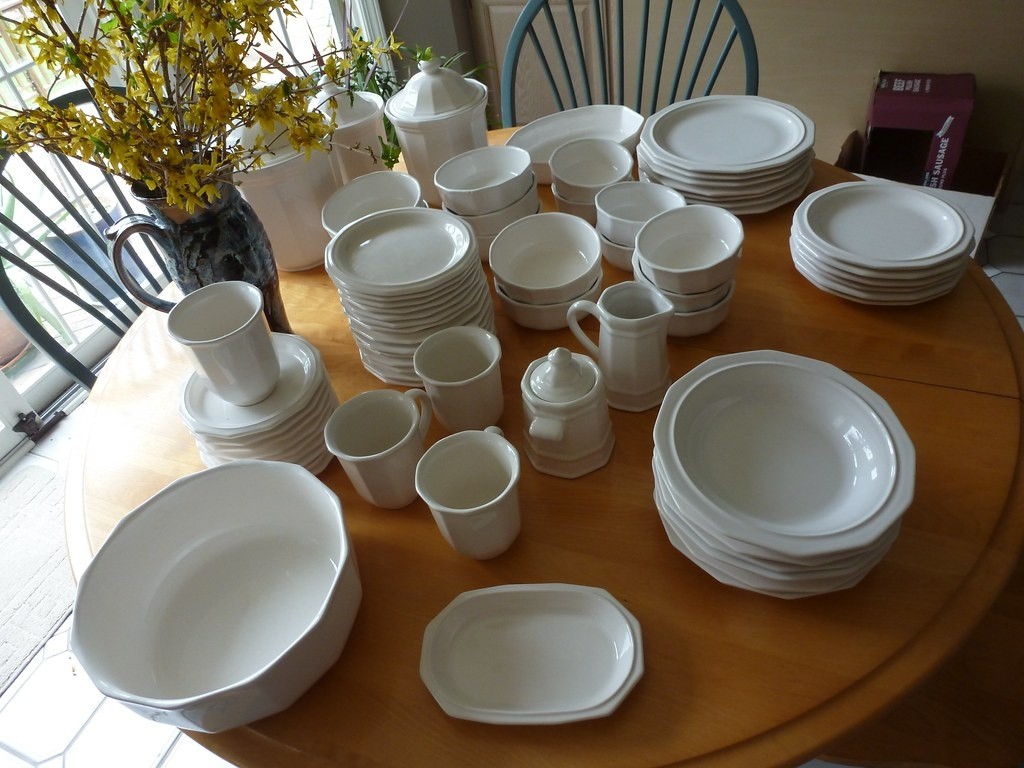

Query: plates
[326,206,496,387]
[652,349,916,600]
[179,332,339,476]
[788,181,975,306]
[420,583,644,725]
[636,94,816,214]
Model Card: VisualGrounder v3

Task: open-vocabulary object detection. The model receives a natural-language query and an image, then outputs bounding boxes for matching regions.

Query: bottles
[226,86,338,273]
[307,74,384,186]
[385,58,488,208]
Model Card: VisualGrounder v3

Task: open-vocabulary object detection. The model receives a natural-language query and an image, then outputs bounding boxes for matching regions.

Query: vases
[40,199,146,299]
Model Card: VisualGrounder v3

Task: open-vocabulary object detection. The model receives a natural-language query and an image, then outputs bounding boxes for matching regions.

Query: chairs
[500,0,758,130]
[0,86,195,393]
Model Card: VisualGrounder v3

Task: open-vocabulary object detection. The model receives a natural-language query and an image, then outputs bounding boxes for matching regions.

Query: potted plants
[1,0,404,335]
[0,168,78,370]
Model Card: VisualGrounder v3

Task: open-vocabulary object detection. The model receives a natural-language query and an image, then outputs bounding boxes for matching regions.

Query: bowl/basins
[71,460,360,734]
[595,180,686,272]
[549,137,634,225]
[506,104,645,184]
[632,205,745,336]
[320,171,429,238]
[490,212,604,330]
[433,144,542,262]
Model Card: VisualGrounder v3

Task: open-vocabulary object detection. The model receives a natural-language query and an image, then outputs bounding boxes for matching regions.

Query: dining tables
[62,126,1024,767]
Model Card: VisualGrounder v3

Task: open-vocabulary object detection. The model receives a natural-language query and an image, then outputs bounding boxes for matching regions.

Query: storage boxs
[859,67,973,190]
[834,126,1009,259]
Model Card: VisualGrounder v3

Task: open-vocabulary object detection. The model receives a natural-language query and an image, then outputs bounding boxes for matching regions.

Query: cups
[167,280,280,407]
[413,324,503,433]
[566,280,674,411]
[415,426,520,559]
[323,389,433,509]
[520,346,615,480]
